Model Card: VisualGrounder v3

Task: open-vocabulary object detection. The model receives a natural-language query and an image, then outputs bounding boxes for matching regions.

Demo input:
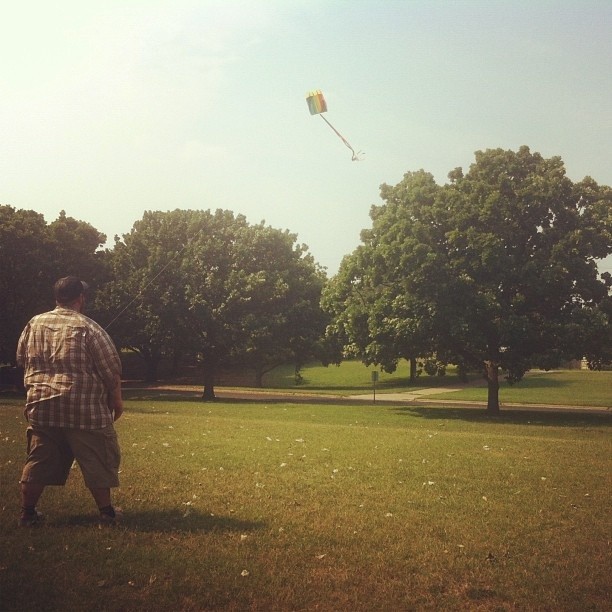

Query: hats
[54,275,88,303]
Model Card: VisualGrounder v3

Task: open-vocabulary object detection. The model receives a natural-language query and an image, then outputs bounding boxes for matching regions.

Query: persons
[15,276,122,526]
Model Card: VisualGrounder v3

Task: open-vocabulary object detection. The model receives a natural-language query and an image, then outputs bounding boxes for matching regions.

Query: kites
[303,90,366,163]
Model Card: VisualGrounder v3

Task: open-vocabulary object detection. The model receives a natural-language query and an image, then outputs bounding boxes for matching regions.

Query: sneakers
[18,511,44,527]
